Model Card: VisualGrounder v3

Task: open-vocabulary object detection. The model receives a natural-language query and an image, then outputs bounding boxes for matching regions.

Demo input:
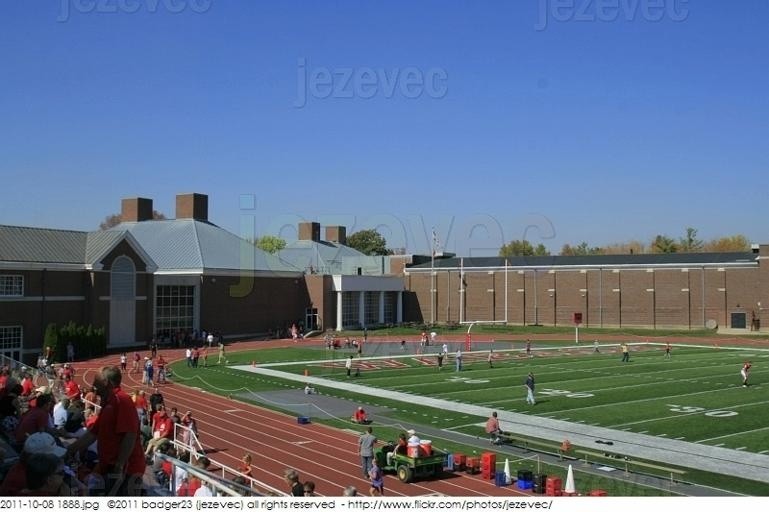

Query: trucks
[372,440,449,482]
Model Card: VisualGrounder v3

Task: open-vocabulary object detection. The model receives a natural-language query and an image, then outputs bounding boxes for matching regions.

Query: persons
[0,324,384,496]
[593,339,600,352]
[488,349,494,368]
[486,411,503,444]
[664,342,672,359]
[741,361,751,388]
[620,344,629,362]
[442,343,448,359]
[525,372,537,405]
[456,350,462,371]
[387,428,420,466]
[437,352,443,367]
[526,339,531,354]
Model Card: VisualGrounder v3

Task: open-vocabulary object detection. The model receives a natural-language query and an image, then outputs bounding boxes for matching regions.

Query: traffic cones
[564,464,577,494]
[501,459,513,484]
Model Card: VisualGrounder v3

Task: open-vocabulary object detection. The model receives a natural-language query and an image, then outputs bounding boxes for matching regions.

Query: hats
[407,429,416,434]
[25,432,67,458]
